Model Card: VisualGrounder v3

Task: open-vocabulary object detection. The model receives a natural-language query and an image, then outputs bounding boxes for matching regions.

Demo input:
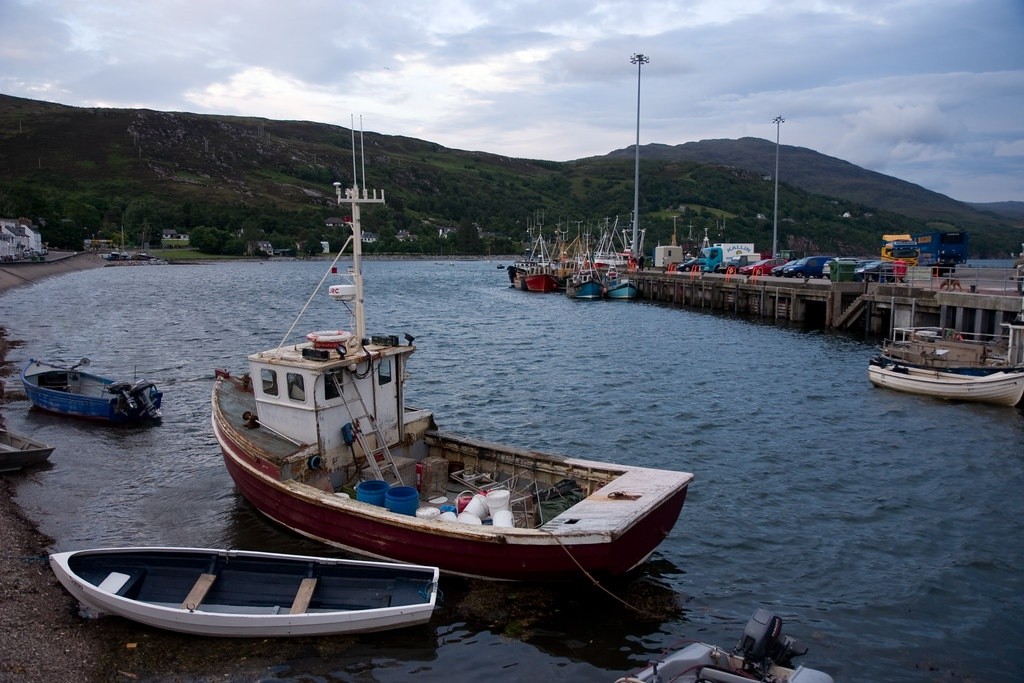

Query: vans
[821,257,861,280]
[782,256,828,279]
[881,232,920,275]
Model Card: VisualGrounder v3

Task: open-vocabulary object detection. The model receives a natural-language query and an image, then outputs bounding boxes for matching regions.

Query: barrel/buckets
[481,518,493,525]
[385,486,420,517]
[463,493,490,519]
[463,474,475,485]
[486,489,511,517]
[416,506,440,520]
[457,511,483,525]
[356,479,390,507]
[969,284,978,293]
[440,512,457,521]
[492,510,515,527]
[448,461,464,483]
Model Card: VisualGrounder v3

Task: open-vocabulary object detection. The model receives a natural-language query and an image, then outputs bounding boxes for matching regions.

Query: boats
[21,358,164,423]
[614,607,834,683]
[49,545,442,639]
[0,428,57,472]
[98,225,158,261]
[866,353,1024,407]
[874,305,1024,371]
[211,112,697,584]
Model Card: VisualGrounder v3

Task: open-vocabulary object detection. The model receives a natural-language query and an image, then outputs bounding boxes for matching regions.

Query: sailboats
[507,208,647,300]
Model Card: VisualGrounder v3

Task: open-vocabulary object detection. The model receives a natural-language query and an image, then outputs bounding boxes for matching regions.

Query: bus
[911,230,970,277]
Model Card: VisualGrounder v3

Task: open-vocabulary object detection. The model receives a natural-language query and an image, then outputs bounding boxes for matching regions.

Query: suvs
[851,259,906,283]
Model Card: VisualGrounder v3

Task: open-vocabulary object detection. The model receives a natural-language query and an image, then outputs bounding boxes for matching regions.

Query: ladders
[451,468,523,499]
[332,369,404,488]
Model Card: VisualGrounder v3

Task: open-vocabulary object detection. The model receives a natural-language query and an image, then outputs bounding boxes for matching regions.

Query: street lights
[629,52,651,264]
[771,113,785,258]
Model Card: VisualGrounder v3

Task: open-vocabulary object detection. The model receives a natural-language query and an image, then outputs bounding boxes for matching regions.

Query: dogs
[940,277,962,291]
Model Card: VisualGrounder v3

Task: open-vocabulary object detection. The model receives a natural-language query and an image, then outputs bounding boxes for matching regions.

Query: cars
[676,255,698,271]
[770,260,800,277]
[743,258,788,276]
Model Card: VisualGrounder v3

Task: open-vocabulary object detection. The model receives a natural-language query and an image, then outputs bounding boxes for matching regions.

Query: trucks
[726,253,761,274]
[697,243,755,274]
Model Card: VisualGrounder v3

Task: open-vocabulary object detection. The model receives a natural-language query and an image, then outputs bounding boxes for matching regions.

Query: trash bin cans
[941,262,956,273]
[829,261,856,282]
